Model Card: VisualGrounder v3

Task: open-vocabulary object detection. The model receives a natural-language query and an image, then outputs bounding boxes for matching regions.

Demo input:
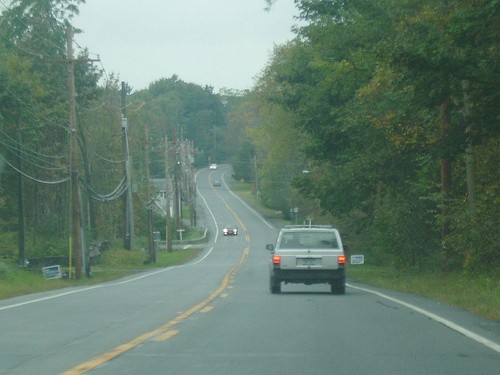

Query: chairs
[286,240,306,248]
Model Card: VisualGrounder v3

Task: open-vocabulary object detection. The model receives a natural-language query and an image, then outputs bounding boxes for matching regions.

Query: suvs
[266,224,347,294]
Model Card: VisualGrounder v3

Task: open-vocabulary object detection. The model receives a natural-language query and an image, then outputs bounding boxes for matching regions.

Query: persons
[293,234,301,244]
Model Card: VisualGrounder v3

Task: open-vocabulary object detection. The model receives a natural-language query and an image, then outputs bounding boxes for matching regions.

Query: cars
[209,164,218,170]
[213,180,220,187]
[222,225,238,235]
